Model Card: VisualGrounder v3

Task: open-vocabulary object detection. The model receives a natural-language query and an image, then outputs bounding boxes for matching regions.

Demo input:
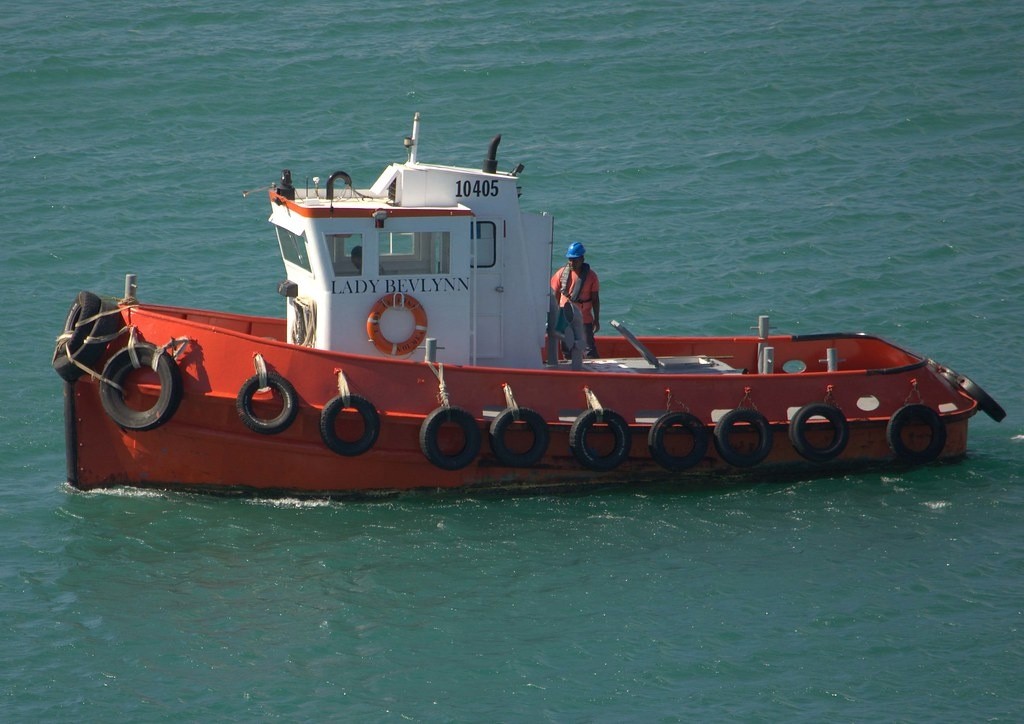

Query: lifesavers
[713,408,776,468]
[318,393,382,458]
[418,405,484,473]
[51,291,125,382]
[647,410,710,474]
[235,371,300,437]
[365,293,429,358]
[787,402,851,466]
[97,340,183,433]
[885,403,948,465]
[567,407,633,471]
[489,406,551,470]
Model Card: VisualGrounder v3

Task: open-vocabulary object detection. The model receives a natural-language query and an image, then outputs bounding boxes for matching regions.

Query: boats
[52,110,978,494]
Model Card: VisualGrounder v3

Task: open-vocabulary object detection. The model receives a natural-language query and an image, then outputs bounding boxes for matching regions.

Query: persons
[551,242,600,359]
[351,246,386,277]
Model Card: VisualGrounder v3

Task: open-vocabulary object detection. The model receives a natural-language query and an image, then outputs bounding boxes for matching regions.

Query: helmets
[565,242,586,258]
